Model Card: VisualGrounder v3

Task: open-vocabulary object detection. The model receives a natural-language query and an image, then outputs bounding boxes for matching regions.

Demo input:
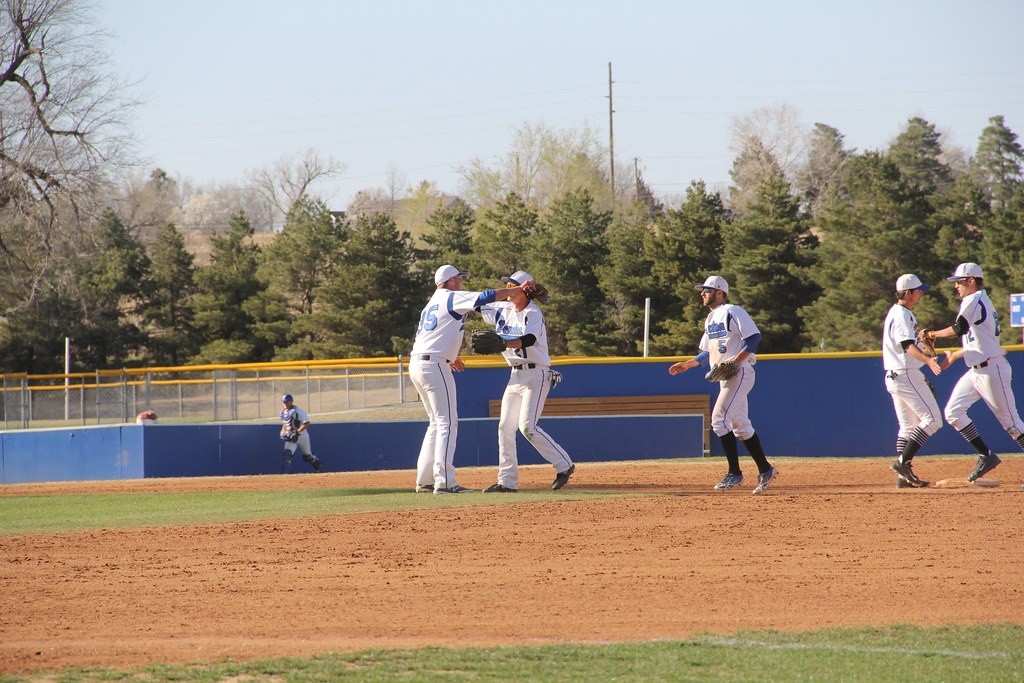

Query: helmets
[282,394,293,403]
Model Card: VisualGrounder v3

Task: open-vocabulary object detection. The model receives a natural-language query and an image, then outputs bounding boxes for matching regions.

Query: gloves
[887,369,898,380]
[548,370,563,390]
[472,329,506,355]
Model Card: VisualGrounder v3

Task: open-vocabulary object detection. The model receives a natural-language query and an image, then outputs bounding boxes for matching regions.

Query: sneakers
[714,470,744,492]
[752,464,780,495]
[414,482,434,492]
[890,459,924,487]
[967,450,1002,481]
[551,463,576,490]
[483,482,518,494]
[433,485,474,494]
[895,476,930,489]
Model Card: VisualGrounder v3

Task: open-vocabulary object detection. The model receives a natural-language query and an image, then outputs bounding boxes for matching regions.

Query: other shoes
[315,465,325,473]
[282,470,293,474]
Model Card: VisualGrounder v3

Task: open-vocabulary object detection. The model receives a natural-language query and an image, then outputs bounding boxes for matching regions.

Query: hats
[895,273,929,290]
[434,264,468,286]
[693,275,729,295]
[501,270,535,296]
[946,262,984,281]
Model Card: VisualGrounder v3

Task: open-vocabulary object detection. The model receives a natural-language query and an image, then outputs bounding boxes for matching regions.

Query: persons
[408,264,534,494]
[471,271,575,493]
[916,263,1024,481]
[882,274,943,488]
[279,394,325,473]
[669,276,778,493]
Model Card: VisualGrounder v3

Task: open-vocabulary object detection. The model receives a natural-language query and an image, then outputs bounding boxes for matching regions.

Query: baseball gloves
[470,330,508,356]
[523,282,551,304]
[286,430,302,443]
[919,329,937,358]
[704,361,740,383]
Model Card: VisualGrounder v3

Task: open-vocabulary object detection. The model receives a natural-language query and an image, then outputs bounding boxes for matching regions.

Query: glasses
[701,288,710,292]
[507,282,517,288]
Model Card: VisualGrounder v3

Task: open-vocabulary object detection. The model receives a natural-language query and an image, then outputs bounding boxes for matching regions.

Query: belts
[417,354,451,364]
[513,363,535,369]
[973,360,988,369]
